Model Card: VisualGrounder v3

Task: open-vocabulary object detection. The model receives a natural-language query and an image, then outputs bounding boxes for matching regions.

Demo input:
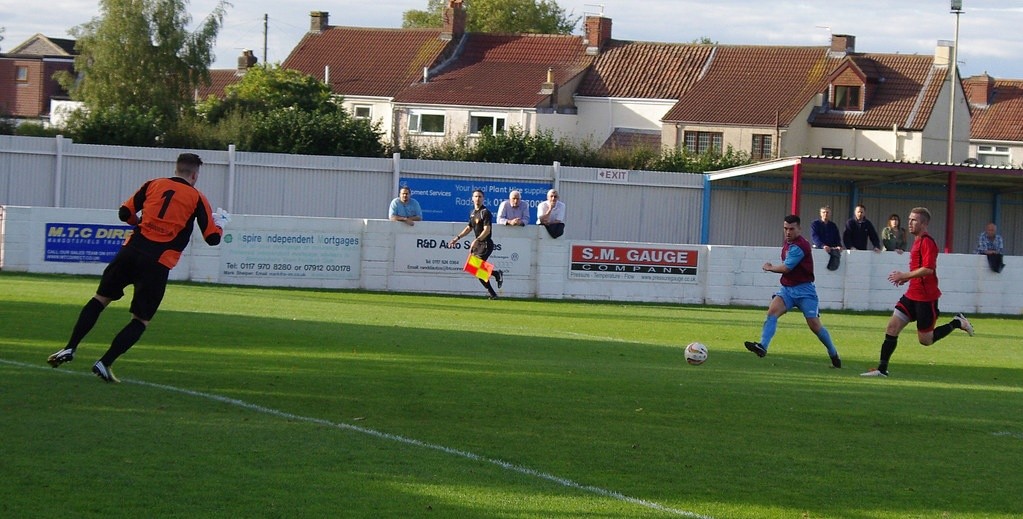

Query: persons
[497,191,530,227]
[976,223,1003,255]
[388,187,422,226]
[47,153,232,383]
[745,215,841,368]
[536,189,565,225]
[842,204,881,253]
[861,207,974,376]
[449,190,503,300]
[810,206,842,253]
[881,214,907,254]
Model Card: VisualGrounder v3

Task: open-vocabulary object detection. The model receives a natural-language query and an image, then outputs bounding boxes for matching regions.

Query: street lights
[949,0,966,166]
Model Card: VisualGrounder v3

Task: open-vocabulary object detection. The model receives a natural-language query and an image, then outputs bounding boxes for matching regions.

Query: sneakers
[826,350,841,369]
[744,340,767,357]
[488,293,499,301]
[496,268,505,287]
[859,369,890,378]
[93,358,121,384]
[46,347,74,367]
[953,312,976,337]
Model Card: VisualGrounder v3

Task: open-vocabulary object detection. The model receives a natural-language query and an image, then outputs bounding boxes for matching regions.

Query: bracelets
[476,238,481,242]
[457,235,461,239]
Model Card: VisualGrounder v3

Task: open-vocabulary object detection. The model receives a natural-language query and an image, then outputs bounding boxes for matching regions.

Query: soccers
[684,342,708,365]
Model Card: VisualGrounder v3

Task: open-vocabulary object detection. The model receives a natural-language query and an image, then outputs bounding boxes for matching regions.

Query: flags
[465,255,494,282]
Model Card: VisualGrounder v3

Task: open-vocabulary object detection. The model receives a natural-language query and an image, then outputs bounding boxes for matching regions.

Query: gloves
[210,206,231,233]
